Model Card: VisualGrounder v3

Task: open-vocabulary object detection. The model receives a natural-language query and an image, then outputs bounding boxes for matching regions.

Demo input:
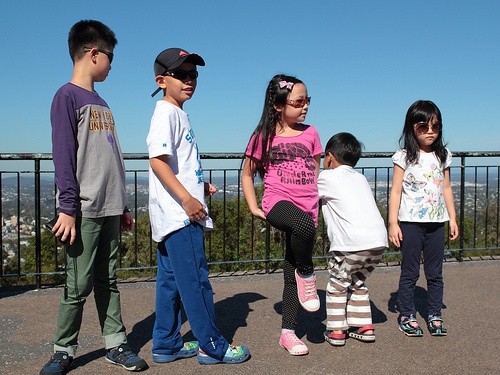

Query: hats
[154,48,206,77]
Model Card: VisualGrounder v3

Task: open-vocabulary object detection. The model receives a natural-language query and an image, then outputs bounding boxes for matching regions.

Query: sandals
[324,329,346,345]
[153,340,199,363]
[348,324,375,341]
[197,344,250,364]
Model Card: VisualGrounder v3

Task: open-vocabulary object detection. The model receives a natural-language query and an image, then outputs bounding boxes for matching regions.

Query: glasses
[415,122,442,134]
[286,96,311,108]
[83,46,113,64]
[163,70,198,79]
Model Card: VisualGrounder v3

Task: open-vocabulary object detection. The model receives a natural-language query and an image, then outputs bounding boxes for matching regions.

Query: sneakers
[39,351,74,375]
[399,314,423,336]
[279,332,309,355]
[295,269,320,312]
[428,316,447,335]
[104,344,148,372]
